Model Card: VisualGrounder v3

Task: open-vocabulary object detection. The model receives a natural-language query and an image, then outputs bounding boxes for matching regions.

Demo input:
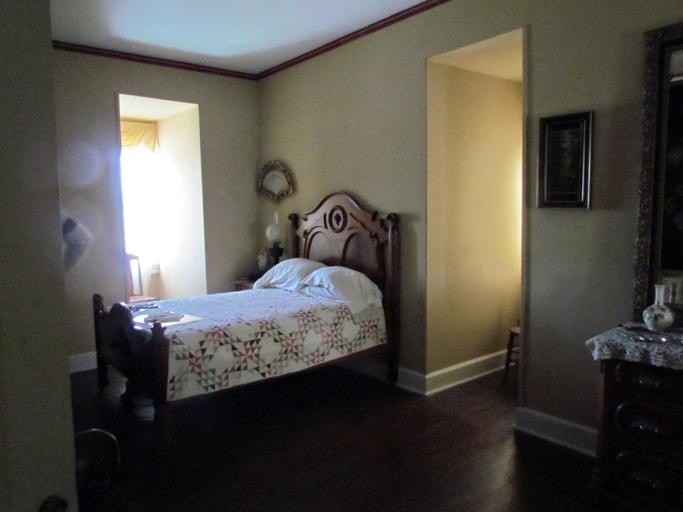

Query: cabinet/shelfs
[598,330,683,512]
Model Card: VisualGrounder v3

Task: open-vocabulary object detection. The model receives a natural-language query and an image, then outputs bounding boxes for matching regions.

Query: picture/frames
[536,110,595,210]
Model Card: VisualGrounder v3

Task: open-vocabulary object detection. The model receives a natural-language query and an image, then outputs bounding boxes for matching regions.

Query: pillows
[253,258,383,301]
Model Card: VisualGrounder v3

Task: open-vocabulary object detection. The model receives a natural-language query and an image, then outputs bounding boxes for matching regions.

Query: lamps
[265,222,290,266]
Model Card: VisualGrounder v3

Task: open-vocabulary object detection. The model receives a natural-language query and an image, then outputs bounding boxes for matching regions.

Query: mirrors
[633,22,683,327]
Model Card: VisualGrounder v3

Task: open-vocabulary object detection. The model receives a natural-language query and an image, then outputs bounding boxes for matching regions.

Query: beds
[92,191,400,453]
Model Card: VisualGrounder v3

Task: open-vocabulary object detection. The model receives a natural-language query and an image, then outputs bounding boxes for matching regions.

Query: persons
[60,209,93,273]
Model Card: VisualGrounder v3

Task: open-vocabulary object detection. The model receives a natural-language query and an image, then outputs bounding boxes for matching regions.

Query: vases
[642,285,677,334]
